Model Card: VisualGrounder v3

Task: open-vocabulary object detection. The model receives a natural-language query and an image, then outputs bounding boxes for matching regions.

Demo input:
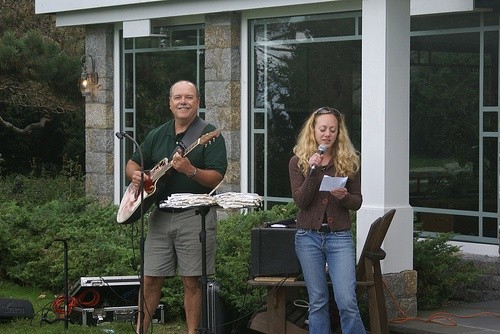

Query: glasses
[317,107,340,117]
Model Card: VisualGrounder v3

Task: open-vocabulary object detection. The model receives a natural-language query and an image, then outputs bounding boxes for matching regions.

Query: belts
[158,207,191,213]
[313,226,338,233]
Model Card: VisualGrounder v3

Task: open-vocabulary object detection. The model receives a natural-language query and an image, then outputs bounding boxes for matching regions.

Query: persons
[125,80,228,334]
[289,106,366,334]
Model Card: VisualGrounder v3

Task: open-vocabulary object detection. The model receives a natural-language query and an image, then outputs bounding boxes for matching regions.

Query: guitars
[116,127,221,225]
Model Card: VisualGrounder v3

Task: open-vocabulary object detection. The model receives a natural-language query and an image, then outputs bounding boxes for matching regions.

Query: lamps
[76,52,101,97]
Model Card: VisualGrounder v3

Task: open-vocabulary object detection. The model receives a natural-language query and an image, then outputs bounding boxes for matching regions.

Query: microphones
[309,143,327,177]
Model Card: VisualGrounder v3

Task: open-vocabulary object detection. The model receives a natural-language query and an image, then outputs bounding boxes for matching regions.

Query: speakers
[0,297,35,324]
[250,218,303,277]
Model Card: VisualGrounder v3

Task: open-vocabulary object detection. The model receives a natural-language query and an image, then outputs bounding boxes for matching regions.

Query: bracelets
[191,168,197,178]
